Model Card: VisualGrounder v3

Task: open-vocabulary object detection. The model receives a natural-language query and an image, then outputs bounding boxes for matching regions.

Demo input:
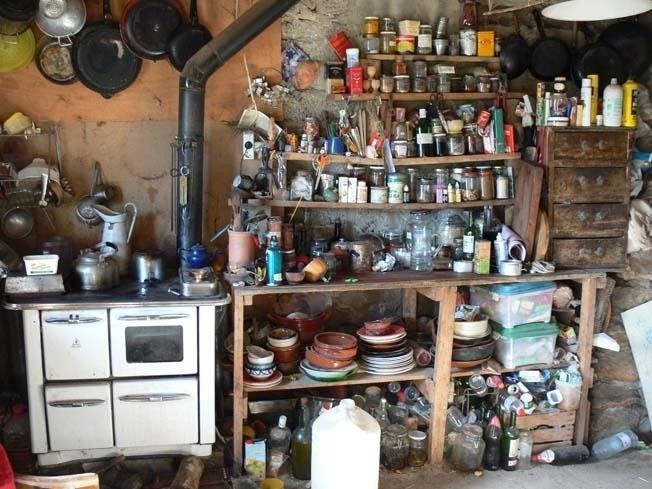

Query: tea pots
[130,246,170,284]
[177,242,221,269]
[72,241,120,292]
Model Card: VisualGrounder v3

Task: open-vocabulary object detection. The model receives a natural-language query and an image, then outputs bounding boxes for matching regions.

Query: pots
[0,206,33,241]
[527,10,572,81]
[34,0,87,48]
[72,1,141,99]
[166,0,214,73]
[571,21,628,95]
[119,0,180,64]
[599,16,652,77]
[33,34,80,86]
[499,8,530,80]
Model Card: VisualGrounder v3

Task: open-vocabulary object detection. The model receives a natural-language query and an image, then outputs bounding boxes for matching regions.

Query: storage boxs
[468,282,556,329]
[496,407,576,457]
[489,319,560,369]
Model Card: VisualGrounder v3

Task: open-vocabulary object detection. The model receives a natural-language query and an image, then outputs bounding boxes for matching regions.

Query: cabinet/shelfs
[225,271,605,478]
[39,307,201,452]
[524,127,633,268]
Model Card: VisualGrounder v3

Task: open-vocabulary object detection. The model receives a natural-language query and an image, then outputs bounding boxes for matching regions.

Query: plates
[356,324,417,376]
[242,373,283,390]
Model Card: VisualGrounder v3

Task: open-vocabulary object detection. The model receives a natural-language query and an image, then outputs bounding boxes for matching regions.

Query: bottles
[461,173,480,201]
[407,430,427,467]
[426,75,438,92]
[493,166,508,199]
[391,141,408,159]
[418,25,432,55]
[310,240,327,257]
[267,415,292,453]
[463,126,483,154]
[485,405,502,470]
[379,16,396,31]
[346,48,359,68]
[291,398,310,480]
[415,109,434,157]
[531,445,589,466]
[459,29,476,56]
[282,224,293,249]
[621,76,639,127]
[430,118,443,134]
[580,78,591,127]
[413,62,427,77]
[476,76,490,92]
[438,74,451,92]
[592,430,639,461]
[431,165,466,203]
[350,167,366,179]
[357,181,367,203]
[362,34,380,54]
[363,16,379,34]
[396,36,415,55]
[376,398,390,432]
[411,76,426,92]
[387,174,409,204]
[415,177,434,202]
[447,366,585,431]
[494,37,500,57]
[379,31,396,54]
[450,77,464,91]
[433,212,504,261]
[447,132,465,155]
[368,166,385,186]
[300,133,308,152]
[330,220,346,252]
[299,228,311,255]
[602,78,623,127]
[393,75,410,93]
[451,424,485,471]
[489,78,499,92]
[501,411,520,470]
[380,424,409,469]
[267,216,282,248]
[432,134,448,156]
[476,166,495,200]
[284,250,297,280]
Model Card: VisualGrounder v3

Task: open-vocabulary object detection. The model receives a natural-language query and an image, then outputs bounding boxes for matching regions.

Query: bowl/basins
[244,323,298,379]
[429,309,495,368]
[295,330,359,382]
